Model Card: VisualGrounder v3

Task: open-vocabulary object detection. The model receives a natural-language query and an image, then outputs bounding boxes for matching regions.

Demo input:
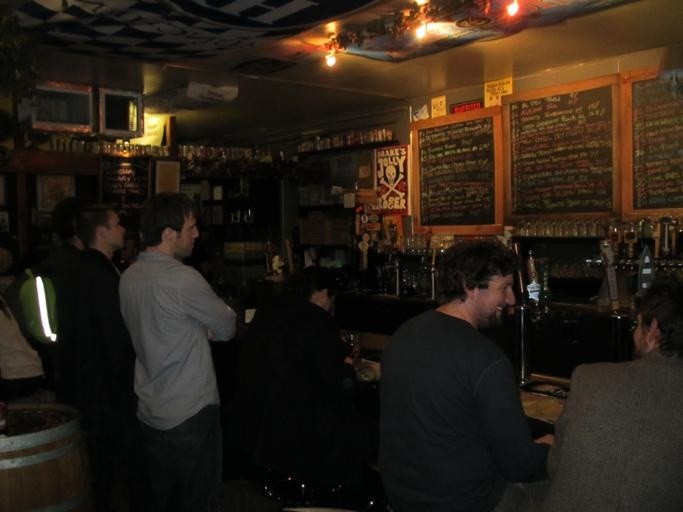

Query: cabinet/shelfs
[222,163,282,266]
[511,237,683,379]
[292,139,397,287]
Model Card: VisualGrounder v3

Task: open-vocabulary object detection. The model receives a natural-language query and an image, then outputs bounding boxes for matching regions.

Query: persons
[119,193,237,512]
[68,207,137,511]
[39,198,86,407]
[547,276,682,511]
[377,238,551,507]
[234,264,358,467]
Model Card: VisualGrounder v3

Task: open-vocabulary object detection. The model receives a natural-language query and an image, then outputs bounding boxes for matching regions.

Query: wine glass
[339,328,361,357]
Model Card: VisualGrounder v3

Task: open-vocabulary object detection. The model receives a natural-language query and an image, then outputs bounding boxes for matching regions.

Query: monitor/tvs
[94,87,145,139]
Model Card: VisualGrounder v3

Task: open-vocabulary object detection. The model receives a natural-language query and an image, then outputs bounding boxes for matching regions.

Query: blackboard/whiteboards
[501,75,621,223]
[409,107,506,235]
[622,68,683,223]
